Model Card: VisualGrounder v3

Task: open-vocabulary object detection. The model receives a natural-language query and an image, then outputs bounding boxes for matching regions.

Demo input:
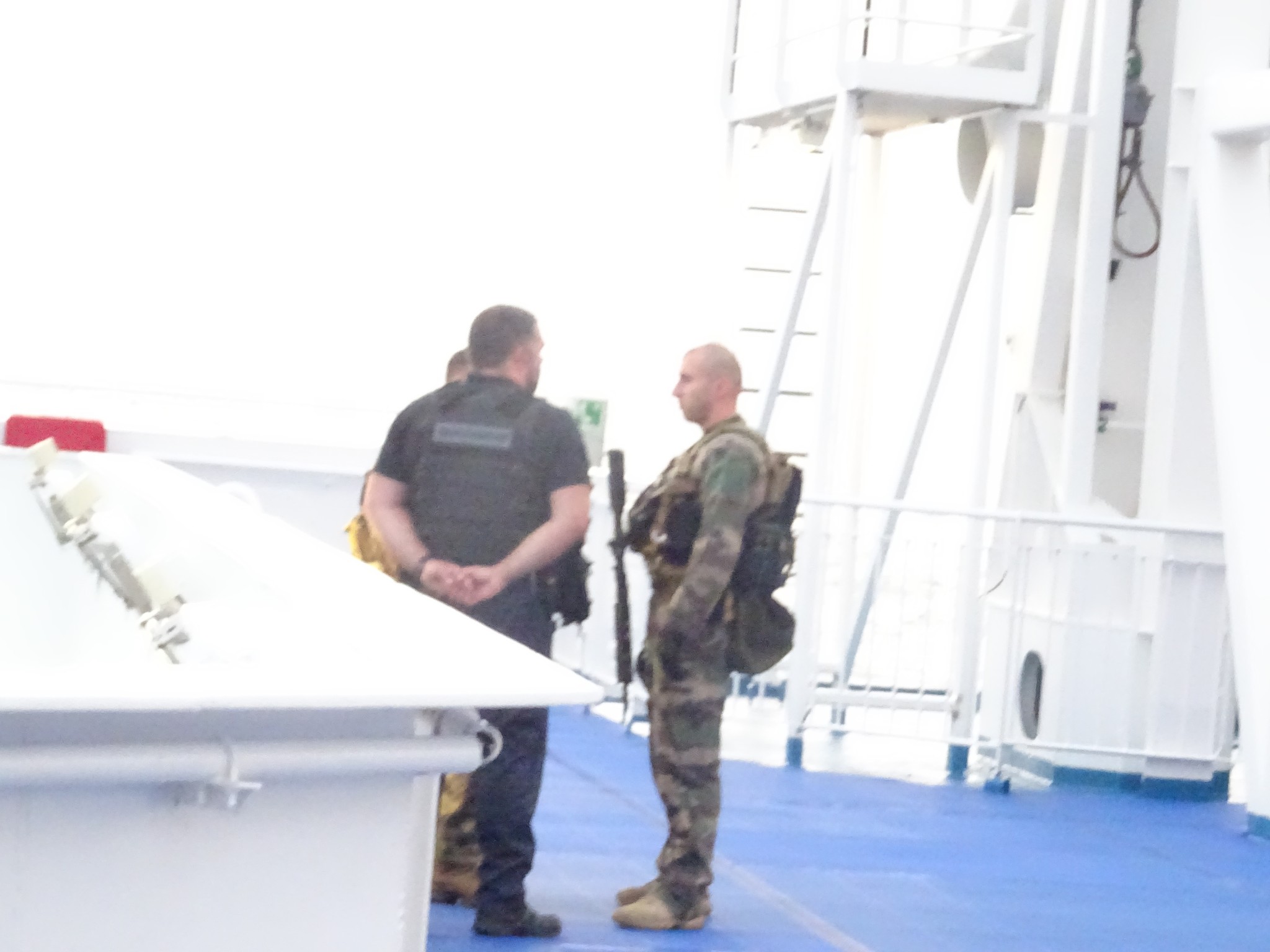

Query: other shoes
[432,867,480,907]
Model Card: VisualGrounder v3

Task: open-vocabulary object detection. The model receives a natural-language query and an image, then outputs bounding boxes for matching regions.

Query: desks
[2,438,609,951]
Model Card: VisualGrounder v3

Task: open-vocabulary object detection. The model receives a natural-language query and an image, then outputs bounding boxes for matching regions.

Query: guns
[607,449,635,712]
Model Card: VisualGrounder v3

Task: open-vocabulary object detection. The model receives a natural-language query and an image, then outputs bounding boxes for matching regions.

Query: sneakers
[474,903,561,935]
[616,878,657,905]
[610,891,706,930]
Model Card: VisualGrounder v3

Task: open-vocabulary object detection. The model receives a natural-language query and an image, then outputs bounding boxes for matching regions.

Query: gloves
[657,629,689,681]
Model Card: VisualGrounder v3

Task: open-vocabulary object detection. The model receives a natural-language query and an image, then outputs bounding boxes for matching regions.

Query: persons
[345,347,501,911]
[611,344,775,928]
[360,306,594,940]
